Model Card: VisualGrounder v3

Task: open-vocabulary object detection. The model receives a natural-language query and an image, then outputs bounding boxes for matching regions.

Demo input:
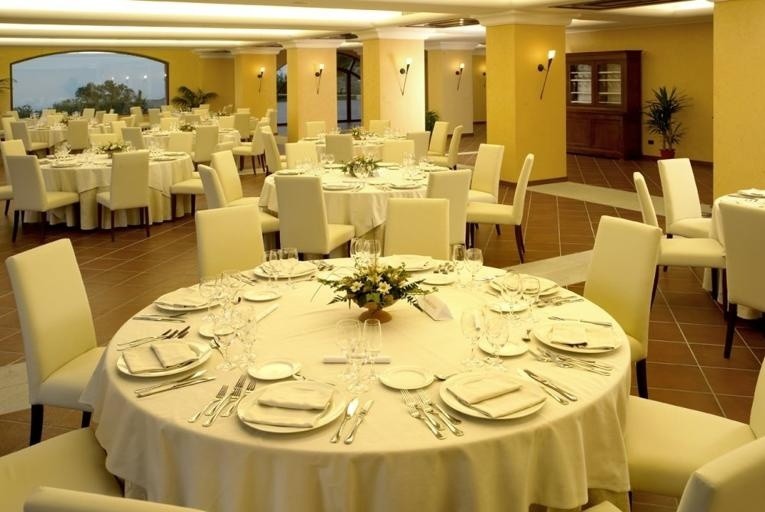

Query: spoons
[241,274,257,281]
[135,369,207,393]
[141,312,188,317]
[533,295,584,308]
[516,368,569,405]
[331,396,359,443]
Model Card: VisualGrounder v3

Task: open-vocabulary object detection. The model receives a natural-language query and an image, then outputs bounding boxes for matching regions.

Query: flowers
[95,141,130,158]
[315,255,438,312]
[351,127,369,141]
[341,154,381,178]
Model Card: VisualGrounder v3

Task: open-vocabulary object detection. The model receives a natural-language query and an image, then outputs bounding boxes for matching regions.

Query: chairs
[1,429,126,512]
[120,116,135,129]
[9,121,50,157]
[233,126,269,176]
[659,158,713,272]
[262,109,276,138]
[96,151,151,242]
[167,133,192,154]
[195,127,219,165]
[672,434,765,512]
[148,109,160,124]
[97,111,106,119]
[5,111,19,121]
[272,175,354,258]
[185,115,200,130]
[263,130,289,171]
[426,169,473,245]
[39,109,57,116]
[614,358,765,511]
[368,120,392,131]
[200,105,211,110]
[2,239,115,445]
[193,109,208,119]
[633,171,732,323]
[113,121,126,133]
[118,128,146,150]
[1,183,13,204]
[222,105,233,113]
[24,481,207,512]
[161,106,175,113]
[431,121,451,156]
[129,107,150,131]
[193,205,266,280]
[386,197,450,261]
[471,143,504,236]
[67,120,89,152]
[198,165,280,250]
[105,114,118,122]
[468,154,535,265]
[170,164,210,220]
[83,108,95,116]
[160,117,179,135]
[304,120,325,140]
[1,140,26,182]
[237,108,250,113]
[1,117,12,140]
[325,134,351,164]
[429,126,463,170]
[406,132,430,164]
[716,201,764,359]
[4,156,79,242]
[237,119,272,146]
[234,113,252,136]
[583,216,663,401]
[207,149,262,206]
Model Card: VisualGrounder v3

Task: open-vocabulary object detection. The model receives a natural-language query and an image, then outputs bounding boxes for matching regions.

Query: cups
[521,277,540,321]
[221,270,241,319]
[460,309,486,368]
[453,245,466,288]
[464,248,483,288]
[216,272,230,315]
[236,304,259,366]
[335,319,361,382]
[350,238,381,269]
[346,338,373,393]
[363,319,382,385]
[281,248,298,288]
[262,251,277,287]
[480,304,503,364]
[501,271,523,321]
[198,276,218,322]
[485,318,510,374]
[213,317,236,371]
[270,249,282,285]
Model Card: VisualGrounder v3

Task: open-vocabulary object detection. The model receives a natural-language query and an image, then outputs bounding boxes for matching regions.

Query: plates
[383,254,435,272]
[246,356,302,381]
[117,340,212,377]
[421,168,449,172]
[738,189,765,198]
[323,182,354,190]
[315,270,357,282]
[489,300,529,313]
[478,335,529,356]
[367,179,385,184]
[153,157,176,161]
[303,137,319,140]
[237,380,346,433]
[490,273,561,296]
[46,154,56,158]
[534,318,622,354]
[199,320,235,338]
[156,290,222,311]
[324,164,346,168]
[379,365,433,390]
[387,183,421,189]
[439,372,547,420]
[244,288,281,301]
[254,261,317,278]
[377,162,400,166]
[165,153,185,156]
[52,163,77,167]
[275,173,300,176]
[419,274,455,285]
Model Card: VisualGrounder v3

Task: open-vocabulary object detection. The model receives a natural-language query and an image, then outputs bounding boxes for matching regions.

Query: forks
[117,326,190,352]
[433,262,454,275]
[205,375,247,416]
[406,403,447,440]
[399,385,440,428]
[415,394,464,436]
[202,387,242,427]
[188,384,229,423]
[416,389,463,425]
[221,378,256,417]
[528,347,612,376]
[309,260,335,271]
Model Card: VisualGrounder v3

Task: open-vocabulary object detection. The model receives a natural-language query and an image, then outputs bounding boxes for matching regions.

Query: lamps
[455,63,464,92]
[316,64,324,94]
[402,58,413,96]
[258,67,265,94]
[538,49,555,100]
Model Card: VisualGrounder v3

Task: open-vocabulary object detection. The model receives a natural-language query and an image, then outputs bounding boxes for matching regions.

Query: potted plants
[641,85,693,158]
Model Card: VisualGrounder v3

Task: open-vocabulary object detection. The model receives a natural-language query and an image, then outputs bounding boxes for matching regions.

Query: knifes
[344,399,375,444]
[523,368,577,401]
[230,274,255,286]
[136,377,216,398]
[132,317,185,323]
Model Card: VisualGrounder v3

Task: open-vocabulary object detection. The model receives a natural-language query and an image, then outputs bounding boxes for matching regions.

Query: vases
[350,294,399,324]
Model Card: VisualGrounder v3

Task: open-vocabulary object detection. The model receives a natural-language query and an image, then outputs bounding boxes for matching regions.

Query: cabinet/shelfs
[568,51,643,159]
